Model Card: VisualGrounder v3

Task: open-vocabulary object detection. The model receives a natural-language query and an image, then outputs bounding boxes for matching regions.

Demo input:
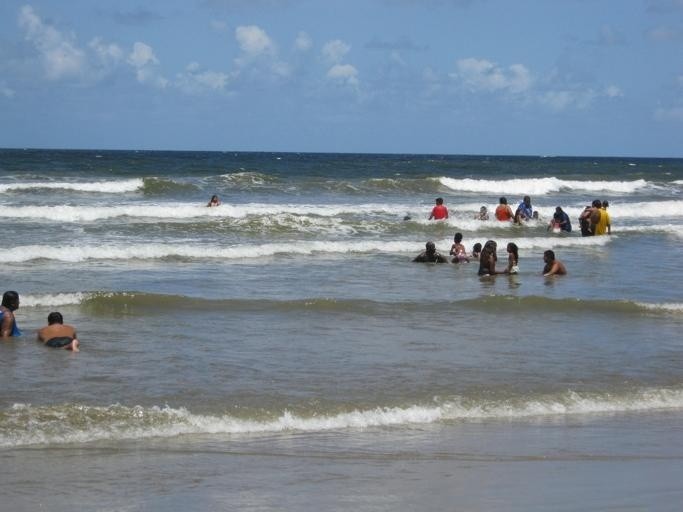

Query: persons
[450,233,469,263]
[473,243,482,257]
[207,195,220,207]
[543,250,566,278]
[0,291,24,337]
[429,198,449,220]
[479,241,497,275]
[37,312,80,353]
[413,241,446,263]
[507,243,520,273]
[475,196,611,237]
